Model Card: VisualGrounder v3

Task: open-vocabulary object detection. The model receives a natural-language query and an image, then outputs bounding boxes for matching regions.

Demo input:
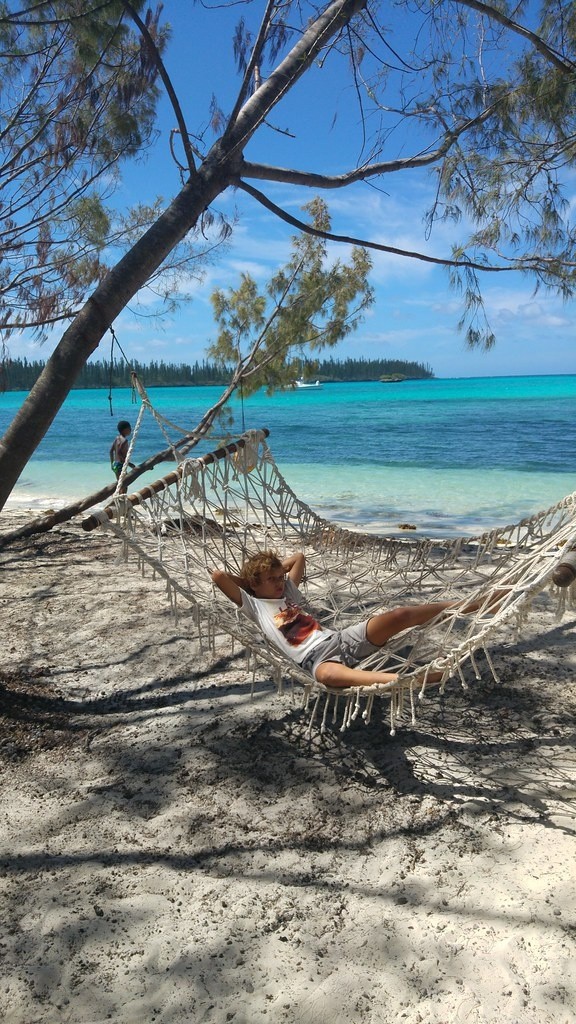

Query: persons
[110,420,136,519]
[212,549,513,687]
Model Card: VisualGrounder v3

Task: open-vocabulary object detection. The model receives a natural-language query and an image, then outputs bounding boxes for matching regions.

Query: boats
[380,378,401,382]
[277,380,323,390]
